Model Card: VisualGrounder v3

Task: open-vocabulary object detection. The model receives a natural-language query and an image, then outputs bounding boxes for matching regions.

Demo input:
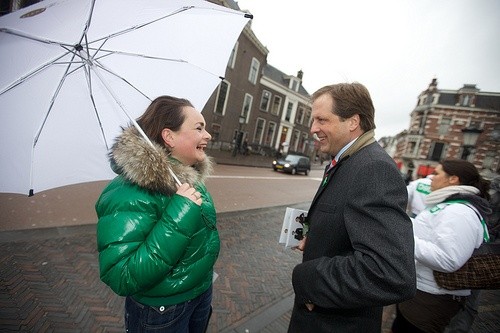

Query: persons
[404,170,413,185]
[292,229,304,241]
[286,82,417,333]
[320,156,324,165]
[390,159,492,333]
[285,229,288,233]
[95,95,220,333]
[406,173,437,219]
[296,213,308,227]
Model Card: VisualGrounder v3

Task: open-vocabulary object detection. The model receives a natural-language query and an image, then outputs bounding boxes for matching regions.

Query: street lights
[232,115,246,158]
[460,126,485,161]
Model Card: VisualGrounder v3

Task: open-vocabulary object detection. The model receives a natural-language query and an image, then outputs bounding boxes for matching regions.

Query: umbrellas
[0,0,254,197]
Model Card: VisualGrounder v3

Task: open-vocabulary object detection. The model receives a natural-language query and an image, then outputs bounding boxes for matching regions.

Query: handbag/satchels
[433,194,500,290]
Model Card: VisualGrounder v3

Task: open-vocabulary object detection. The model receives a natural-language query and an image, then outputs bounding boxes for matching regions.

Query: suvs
[272,155,311,176]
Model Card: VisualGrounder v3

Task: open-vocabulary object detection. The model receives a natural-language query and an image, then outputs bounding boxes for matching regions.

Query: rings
[193,193,199,200]
